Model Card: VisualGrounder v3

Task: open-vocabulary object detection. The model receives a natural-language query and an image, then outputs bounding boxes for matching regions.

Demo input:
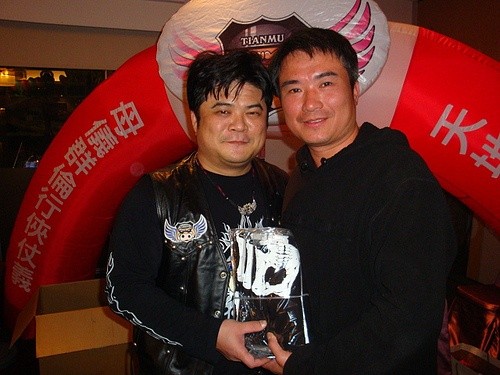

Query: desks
[450,284,500,351]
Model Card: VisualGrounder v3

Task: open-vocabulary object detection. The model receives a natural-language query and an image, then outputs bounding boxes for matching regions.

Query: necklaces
[196,159,257,215]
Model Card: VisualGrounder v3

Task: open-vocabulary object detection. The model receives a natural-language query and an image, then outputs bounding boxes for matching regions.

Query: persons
[262,29,456,375]
[104,50,291,375]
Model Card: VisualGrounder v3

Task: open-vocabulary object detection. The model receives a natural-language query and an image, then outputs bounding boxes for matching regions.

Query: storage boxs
[7,278,140,375]
